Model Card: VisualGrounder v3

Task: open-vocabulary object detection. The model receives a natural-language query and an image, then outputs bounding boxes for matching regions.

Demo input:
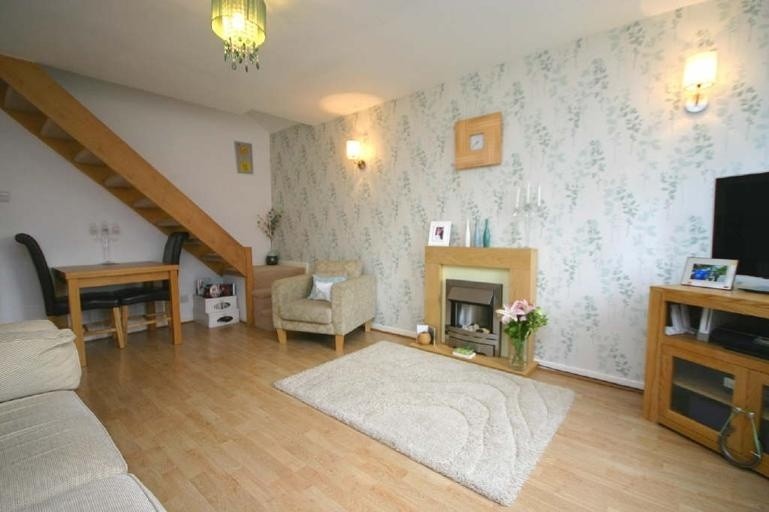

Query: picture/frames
[679,254,743,292]
[427,220,453,248]
[233,140,255,174]
[453,112,505,171]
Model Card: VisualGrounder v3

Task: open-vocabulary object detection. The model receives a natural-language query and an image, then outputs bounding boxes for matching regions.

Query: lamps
[209,0,269,74]
[346,139,366,170]
[672,48,717,114]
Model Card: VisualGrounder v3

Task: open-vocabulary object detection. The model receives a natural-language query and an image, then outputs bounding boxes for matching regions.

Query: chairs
[119,229,190,346]
[14,232,126,351]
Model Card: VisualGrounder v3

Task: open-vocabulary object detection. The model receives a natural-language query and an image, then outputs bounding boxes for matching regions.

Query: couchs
[0,317,167,512]
[270,257,376,352]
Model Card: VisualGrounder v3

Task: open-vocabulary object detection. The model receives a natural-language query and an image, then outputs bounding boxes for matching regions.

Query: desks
[50,260,184,366]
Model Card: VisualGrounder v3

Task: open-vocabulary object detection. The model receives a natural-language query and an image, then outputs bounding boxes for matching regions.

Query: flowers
[494,297,548,365]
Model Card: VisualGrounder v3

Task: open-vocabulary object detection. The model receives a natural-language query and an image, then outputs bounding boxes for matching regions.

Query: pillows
[307,270,344,301]
[313,280,333,301]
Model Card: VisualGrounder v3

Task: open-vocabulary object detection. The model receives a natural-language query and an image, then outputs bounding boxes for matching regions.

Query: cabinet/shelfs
[640,279,769,481]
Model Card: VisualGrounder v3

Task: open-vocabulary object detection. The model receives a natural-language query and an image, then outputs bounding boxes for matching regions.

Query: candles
[514,188,520,209]
[538,184,542,208]
[526,184,531,206]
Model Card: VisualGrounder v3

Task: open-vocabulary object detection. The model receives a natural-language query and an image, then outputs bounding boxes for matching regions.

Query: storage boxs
[193,292,237,314]
[193,308,241,329]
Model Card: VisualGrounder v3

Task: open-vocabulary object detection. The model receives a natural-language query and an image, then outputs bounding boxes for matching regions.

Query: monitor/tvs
[711,171,768,292]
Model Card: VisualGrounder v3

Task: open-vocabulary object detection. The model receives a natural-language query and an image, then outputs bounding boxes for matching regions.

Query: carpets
[272,341,580,506]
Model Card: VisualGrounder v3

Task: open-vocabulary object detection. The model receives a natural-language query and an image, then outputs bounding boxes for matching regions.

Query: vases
[475,219,481,247]
[416,330,433,346]
[465,219,472,248]
[510,337,528,371]
[483,218,492,249]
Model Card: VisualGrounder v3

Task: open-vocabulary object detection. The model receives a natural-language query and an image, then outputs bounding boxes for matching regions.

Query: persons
[436,227,443,239]
[708,270,719,282]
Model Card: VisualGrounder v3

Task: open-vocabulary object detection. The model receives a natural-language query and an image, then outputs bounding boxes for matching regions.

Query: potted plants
[257,205,283,267]
